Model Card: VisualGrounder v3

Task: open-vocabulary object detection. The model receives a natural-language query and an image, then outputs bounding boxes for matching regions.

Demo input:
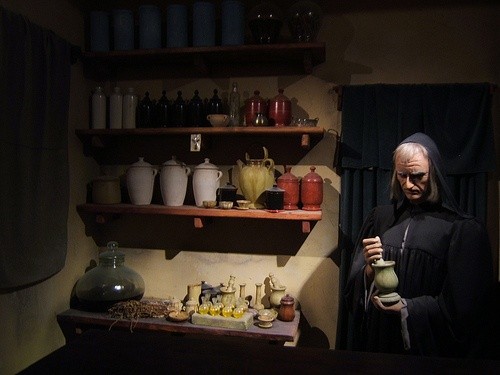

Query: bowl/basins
[208,114,230,127]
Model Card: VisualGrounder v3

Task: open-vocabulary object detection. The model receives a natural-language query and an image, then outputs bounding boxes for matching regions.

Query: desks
[57,306,302,345]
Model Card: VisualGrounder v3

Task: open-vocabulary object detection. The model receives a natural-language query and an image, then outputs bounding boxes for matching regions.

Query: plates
[258,309,278,320]
[169,312,189,322]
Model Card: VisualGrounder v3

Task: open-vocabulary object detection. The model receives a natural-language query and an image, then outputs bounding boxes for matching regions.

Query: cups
[93,175,121,205]
[189,285,202,307]
[276,167,300,210]
[215,186,238,206]
[371,261,400,303]
[265,184,286,210]
[218,201,234,208]
[278,293,295,322]
[237,200,252,209]
[169,296,181,312]
[159,156,190,207]
[192,158,223,208]
[301,166,323,211]
[126,157,155,206]
[257,315,274,328]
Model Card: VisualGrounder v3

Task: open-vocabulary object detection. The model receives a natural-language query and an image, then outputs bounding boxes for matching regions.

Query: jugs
[236,146,275,210]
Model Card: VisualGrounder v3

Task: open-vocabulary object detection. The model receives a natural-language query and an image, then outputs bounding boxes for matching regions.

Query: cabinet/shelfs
[75,42,325,232]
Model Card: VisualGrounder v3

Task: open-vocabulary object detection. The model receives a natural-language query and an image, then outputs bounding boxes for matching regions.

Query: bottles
[90,85,291,128]
[89,0,320,53]
[74,241,145,313]
[220,286,236,306]
[240,283,249,307]
[253,283,264,312]
[270,287,287,311]
[198,295,248,319]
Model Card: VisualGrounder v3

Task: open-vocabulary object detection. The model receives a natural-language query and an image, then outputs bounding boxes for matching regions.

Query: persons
[343,133,500,375]
